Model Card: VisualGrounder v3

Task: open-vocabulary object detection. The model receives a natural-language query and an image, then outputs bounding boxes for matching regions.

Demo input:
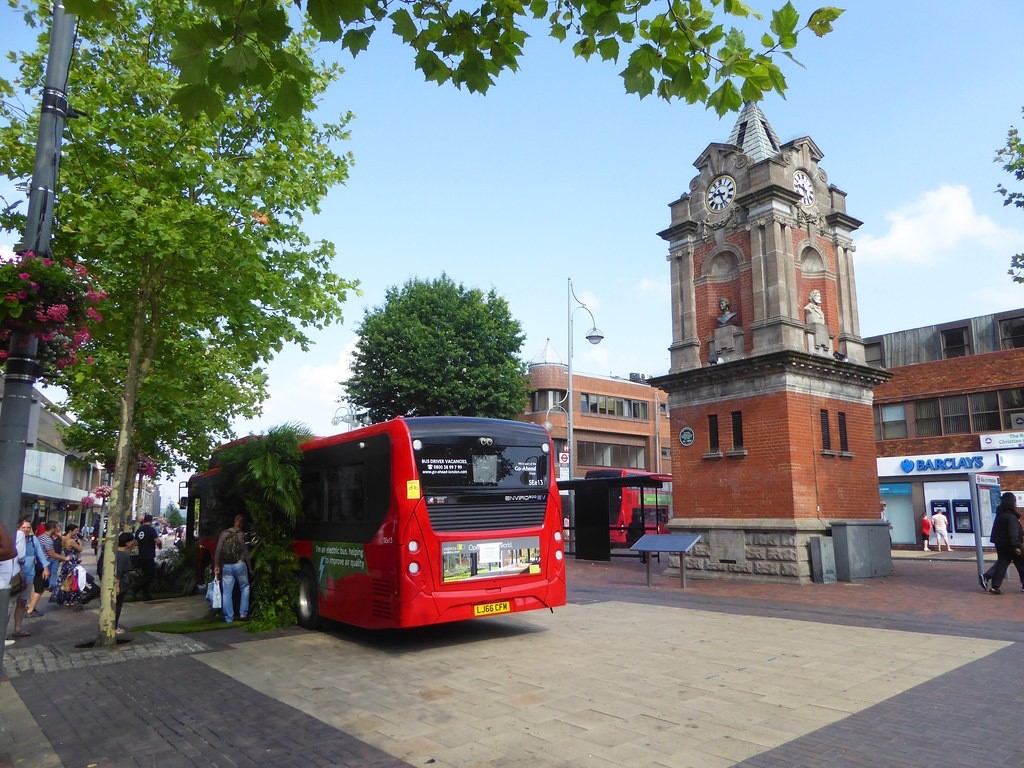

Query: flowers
[56,502,67,512]
[103,463,117,473]
[81,496,95,505]
[0,252,110,376]
[67,504,78,511]
[92,485,115,498]
[137,453,158,477]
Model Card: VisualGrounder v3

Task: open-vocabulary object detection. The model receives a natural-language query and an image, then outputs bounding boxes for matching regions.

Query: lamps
[707,355,718,367]
[834,350,845,361]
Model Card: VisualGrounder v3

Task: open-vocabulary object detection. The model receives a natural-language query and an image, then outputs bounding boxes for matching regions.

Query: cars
[155,523,187,552]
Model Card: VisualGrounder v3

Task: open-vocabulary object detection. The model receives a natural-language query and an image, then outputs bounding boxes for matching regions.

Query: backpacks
[222,529,242,564]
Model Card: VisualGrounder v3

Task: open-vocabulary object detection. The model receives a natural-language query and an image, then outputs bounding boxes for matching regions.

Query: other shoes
[24,606,40,613]
[115,628,125,634]
[1021,586,1024,591]
[989,587,1004,594]
[4,639,15,646]
[979,574,987,590]
[948,548,954,552]
[16,630,31,636]
[240,614,246,619]
[25,611,43,618]
[143,596,155,600]
[924,548,931,551]
[128,589,136,601]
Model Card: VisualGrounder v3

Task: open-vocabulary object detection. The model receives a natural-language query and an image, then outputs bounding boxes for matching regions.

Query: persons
[977,492,1024,597]
[880,501,892,550]
[97,532,142,635]
[921,512,932,552]
[717,298,737,326]
[804,289,825,325]
[213,513,254,624]
[10,517,82,638]
[0,523,18,563]
[81,515,191,552]
[931,509,954,551]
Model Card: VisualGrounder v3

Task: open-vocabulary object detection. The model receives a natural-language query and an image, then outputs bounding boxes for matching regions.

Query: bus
[582,467,673,550]
[177,415,568,633]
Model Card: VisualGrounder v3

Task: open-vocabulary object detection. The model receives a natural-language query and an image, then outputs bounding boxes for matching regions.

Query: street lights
[331,406,373,432]
[566,276,606,557]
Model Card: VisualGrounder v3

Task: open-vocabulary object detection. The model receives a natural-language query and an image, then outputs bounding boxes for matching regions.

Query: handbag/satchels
[10,570,27,597]
[32,536,49,587]
[206,574,221,608]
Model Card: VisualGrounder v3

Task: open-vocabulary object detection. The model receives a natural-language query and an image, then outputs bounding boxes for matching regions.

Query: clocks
[705,174,737,214]
[792,170,817,207]
